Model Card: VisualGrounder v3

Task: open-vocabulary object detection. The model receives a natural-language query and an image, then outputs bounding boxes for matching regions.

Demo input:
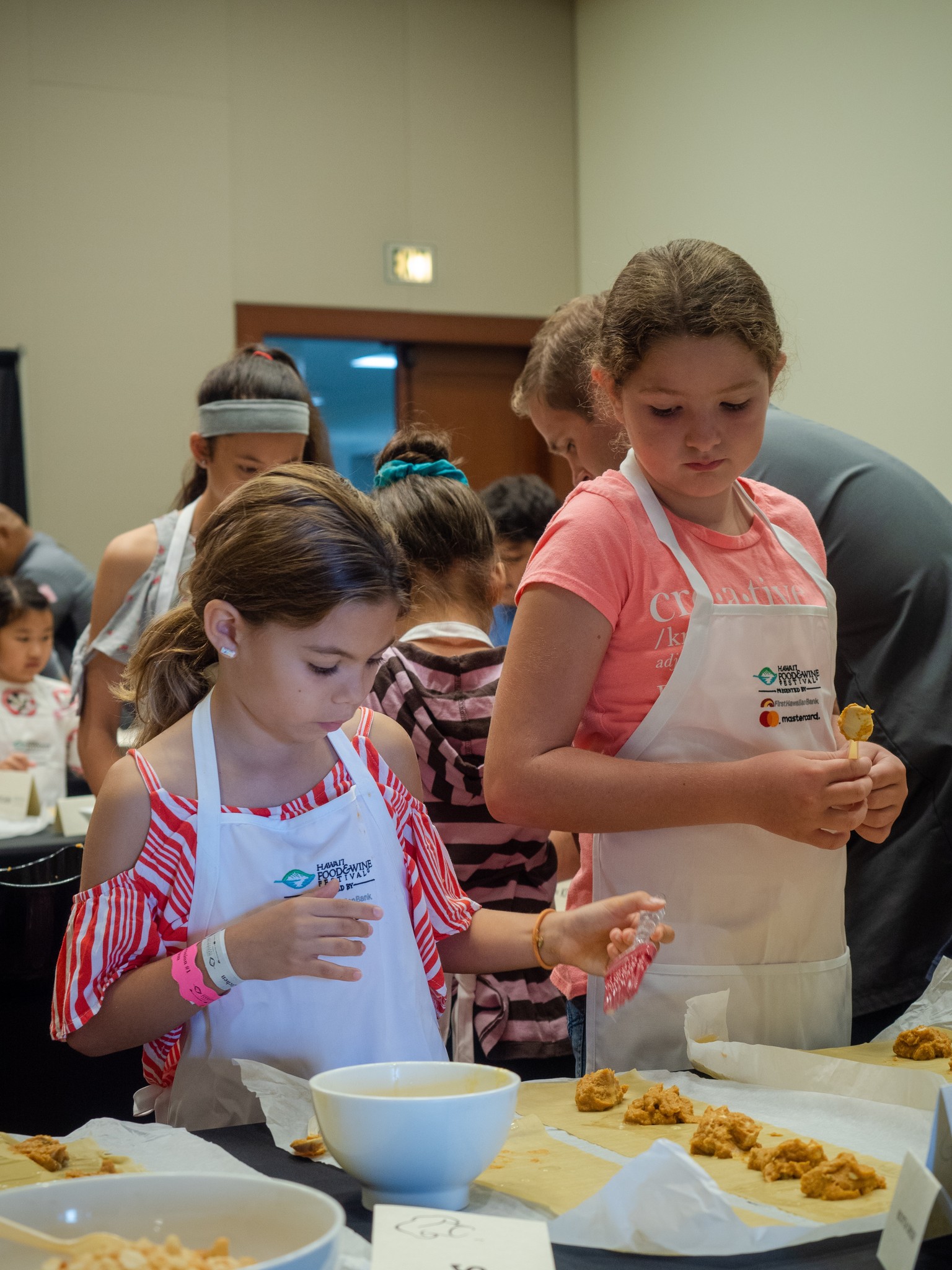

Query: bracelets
[169,941,231,1008]
[531,908,557,970]
[198,927,244,993]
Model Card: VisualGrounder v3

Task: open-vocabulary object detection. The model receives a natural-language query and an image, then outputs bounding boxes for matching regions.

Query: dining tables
[0,1028,952,1270]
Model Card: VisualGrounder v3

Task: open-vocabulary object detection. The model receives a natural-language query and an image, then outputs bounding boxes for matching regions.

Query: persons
[500,279,952,1048]
[481,239,913,1086]
[0,577,86,812]
[352,422,579,1086]
[1,503,98,685]
[468,475,562,649]
[42,455,680,1145]
[73,337,335,798]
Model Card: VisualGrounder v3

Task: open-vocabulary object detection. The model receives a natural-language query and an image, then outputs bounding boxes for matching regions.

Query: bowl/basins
[0,1170,345,1270]
[307,1062,523,1214]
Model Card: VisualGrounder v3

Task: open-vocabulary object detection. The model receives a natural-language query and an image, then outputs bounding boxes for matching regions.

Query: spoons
[839,704,873,761]
[1,1215,121,1257]
